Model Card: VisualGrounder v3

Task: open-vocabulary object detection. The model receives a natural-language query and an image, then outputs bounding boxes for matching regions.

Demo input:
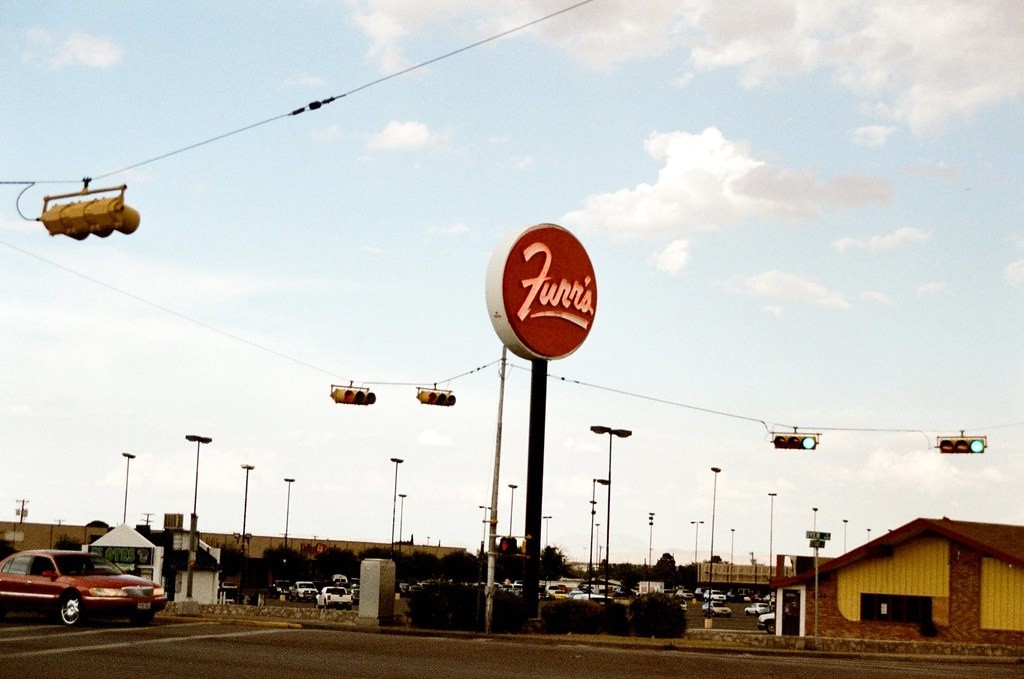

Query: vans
[332,574,348,584]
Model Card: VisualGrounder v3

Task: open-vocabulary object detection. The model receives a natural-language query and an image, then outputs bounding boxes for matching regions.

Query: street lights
[691,521,704,563]
[392,458,404,559]
[768,493,778,613]
[843,520,848,554]
[588,479,610,601]
[283,479,295,549]
[237,466,254,604]
[730,529,735,582]
[396,494,407,593]
[705,467,722,629]
[176,435,213,615]
[427,537,430,545]
[812,508,818,531]
[121,453,136,523]
[867,529,871,542]
[590,426,632,610]
[479,506,492,541]
[648,513,656,593]
[508,484,518,536]
[543,517,552,548]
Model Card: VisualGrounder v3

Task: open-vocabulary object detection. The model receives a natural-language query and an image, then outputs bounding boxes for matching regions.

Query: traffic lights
[330,389,376,406]
[498,537,517,554]
[416,391,456,406]
[772,435,819,450]
[39,197,140,241]
[936,439,987,454]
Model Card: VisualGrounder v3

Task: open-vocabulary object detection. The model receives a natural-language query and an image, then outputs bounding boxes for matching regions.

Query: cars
[397,579,439,597]
[662,589,732,617]
[539,584,637,605]
[0,549,168,627]
[346,578,360,604]
[462,580,523,597]
[727,591,775,602]
[757,612,775,634]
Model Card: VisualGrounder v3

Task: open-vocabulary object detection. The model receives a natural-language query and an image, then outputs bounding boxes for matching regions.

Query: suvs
[745,603,770,616]
[270,580,318,602]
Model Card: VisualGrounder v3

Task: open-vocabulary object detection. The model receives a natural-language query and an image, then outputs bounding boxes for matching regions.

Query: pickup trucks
[315,587,355,611]
[703,590,726,602]
